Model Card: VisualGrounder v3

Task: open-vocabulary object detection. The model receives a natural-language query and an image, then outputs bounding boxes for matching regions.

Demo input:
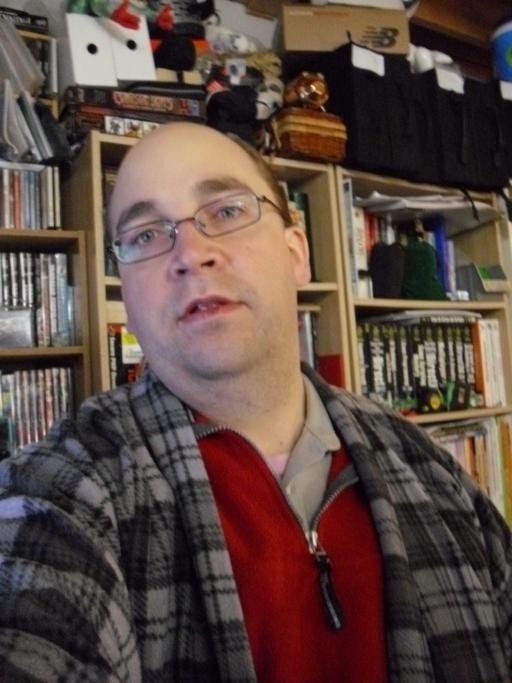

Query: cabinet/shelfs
[1,10,93,453]
[63,127,355,406]
[336,167,511,535]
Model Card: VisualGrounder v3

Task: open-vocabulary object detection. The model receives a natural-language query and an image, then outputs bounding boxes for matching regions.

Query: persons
[0,117,511,683]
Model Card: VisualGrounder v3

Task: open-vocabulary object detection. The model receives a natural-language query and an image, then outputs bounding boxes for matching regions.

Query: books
[280,179,343,395]
[3,252,82,348]
[108,89,208,118]
[2,366,75,457]
[1,5,74,230]
[122,80,208,101]
[417,417,512,526]
[344,177,508,301]
[353,308,507,413]
[104,168,146,392]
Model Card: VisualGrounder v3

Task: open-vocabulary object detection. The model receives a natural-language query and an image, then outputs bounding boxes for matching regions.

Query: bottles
[402,206,443,299]
[367,209,405,298]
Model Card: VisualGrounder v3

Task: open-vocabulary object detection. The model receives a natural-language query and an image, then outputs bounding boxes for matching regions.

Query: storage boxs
[54,12,119,96]
[105,13,159,90]
[268,3,410,62]
[268,105,346,168]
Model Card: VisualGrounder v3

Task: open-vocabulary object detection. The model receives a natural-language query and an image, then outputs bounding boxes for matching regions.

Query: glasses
[109,190,289,266]
